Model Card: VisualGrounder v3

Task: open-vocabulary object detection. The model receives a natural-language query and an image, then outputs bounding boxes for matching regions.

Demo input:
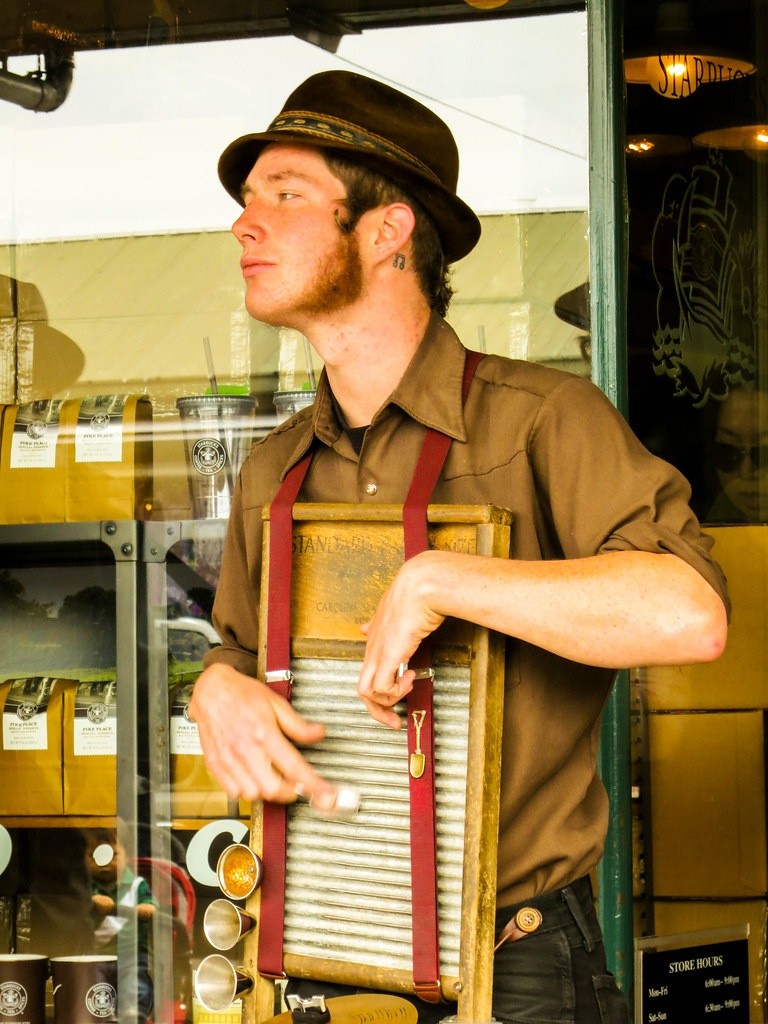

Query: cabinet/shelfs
[0,520,136,1024]
[143,519,244,1024]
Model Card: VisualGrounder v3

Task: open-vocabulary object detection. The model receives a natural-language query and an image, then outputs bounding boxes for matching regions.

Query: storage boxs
[0,890,116,958]
[0,393,153,524]
[627,525,768,1024]
[0,683,247,814]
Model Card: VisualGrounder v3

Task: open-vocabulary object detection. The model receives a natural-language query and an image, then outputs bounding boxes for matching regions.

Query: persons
[706,373,768,526]
[187,71,729,1024]
[87,827,159,1024]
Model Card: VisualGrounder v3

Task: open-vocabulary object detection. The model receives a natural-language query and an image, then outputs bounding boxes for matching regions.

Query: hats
[216,69,484,264]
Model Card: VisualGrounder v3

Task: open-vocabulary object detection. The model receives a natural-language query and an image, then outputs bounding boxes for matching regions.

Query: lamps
[623,0,756,100]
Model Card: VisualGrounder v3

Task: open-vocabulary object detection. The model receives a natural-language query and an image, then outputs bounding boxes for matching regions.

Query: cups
[271,389,317,426]
[177,394,259,520]
[50,955,118,1024]
[0,953,50,1024]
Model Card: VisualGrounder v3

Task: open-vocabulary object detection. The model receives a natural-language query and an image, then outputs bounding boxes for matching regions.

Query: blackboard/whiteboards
[634,921,750,1024]
